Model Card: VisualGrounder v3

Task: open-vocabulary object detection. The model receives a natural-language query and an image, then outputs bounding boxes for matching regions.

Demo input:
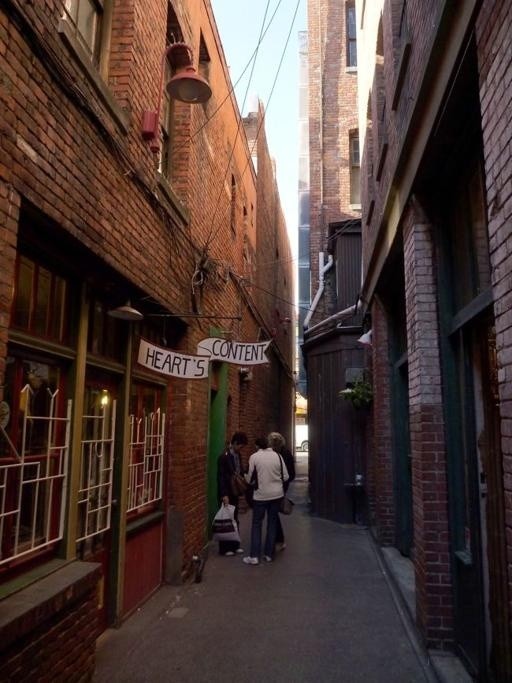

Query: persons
[269,432,296,552]
[243,436,290,566]
[215,430,250,556]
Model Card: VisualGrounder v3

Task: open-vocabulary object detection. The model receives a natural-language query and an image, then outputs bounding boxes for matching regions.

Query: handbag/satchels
[250,465,259,491]
[277,496,293,515]
[229,471,247,495]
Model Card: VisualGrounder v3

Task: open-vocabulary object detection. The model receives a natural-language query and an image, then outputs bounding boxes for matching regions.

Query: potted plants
[338,367,373,421]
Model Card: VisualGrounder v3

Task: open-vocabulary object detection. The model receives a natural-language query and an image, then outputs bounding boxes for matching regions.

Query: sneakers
[225,544,287,564]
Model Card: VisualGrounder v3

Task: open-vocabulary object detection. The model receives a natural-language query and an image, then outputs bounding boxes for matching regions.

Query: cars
[295,424,308,452]
